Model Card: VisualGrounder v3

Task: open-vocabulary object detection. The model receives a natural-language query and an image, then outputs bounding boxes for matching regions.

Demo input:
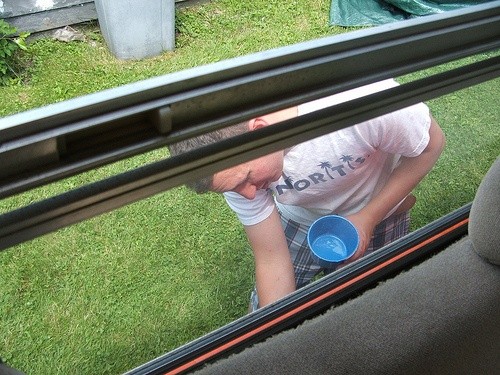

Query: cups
[307,214,361,271]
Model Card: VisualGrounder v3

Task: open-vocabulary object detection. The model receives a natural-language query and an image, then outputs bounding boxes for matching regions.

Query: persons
[166,78,445,315]
